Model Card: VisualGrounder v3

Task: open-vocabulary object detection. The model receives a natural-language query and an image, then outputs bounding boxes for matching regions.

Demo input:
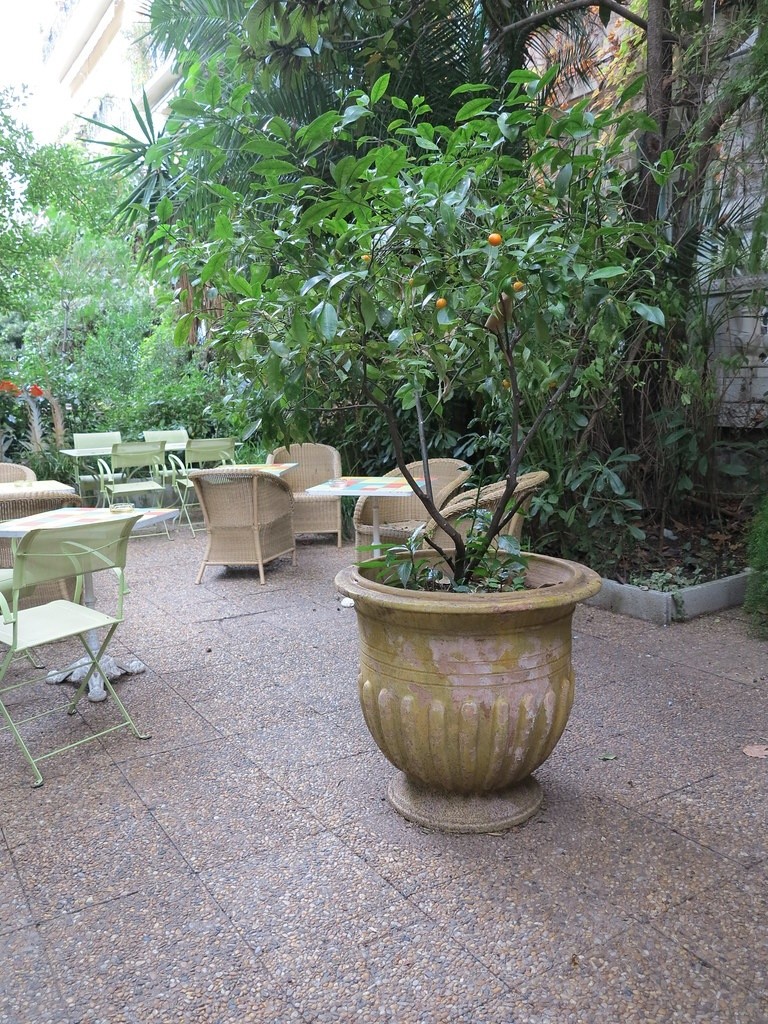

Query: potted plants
[121,44,704,835]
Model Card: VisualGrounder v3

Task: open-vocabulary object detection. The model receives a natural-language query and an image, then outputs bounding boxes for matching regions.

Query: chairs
[187,470,297,584]
[353,458,473,566]
[264,443,343,547]
[421,471,550,550]
[0,494,85,614]
[0,514,153,788]
[0,569,45,670]
[74,429,237,541]
[0,462,37,484]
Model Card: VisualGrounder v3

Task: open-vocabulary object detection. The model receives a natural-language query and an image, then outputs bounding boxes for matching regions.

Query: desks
[0,507,181,702]
[1,480,75,494]
[59,442,243,507]
[305,476,439,608]
[211,463,299,477]
